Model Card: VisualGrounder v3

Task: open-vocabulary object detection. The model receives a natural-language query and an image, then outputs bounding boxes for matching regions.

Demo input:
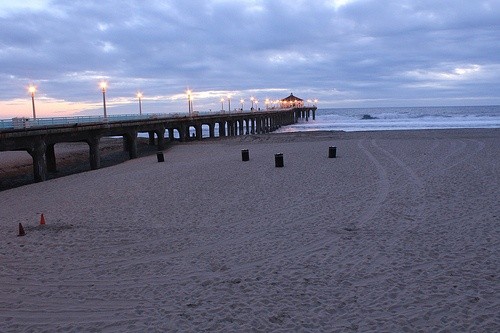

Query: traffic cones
[39,214,45,224]
[17,223,26,236]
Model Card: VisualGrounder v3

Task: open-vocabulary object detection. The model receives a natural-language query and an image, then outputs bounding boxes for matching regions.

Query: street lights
[186,89,192,112]
[99,81,108,116]
[221,98,224,109]
[138,93,142,115]
[241,99,244,109]
[226,94,232,111]
[250,97,318,112]
[28,87,36,118]
